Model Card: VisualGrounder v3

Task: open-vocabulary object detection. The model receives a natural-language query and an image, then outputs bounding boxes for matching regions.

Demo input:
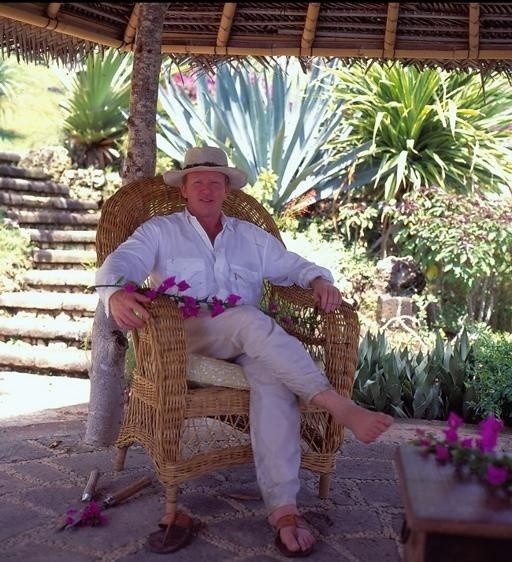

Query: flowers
[415,409,512,495]
[65,498,108,528]
[88,274,310,328]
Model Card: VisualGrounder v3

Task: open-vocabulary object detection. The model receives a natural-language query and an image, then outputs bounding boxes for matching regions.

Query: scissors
[55,468,152,533]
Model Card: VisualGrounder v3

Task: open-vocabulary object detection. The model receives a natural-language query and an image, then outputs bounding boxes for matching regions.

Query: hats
[163,147,247,194]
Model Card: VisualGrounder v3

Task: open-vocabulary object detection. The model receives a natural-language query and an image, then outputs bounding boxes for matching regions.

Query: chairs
[94,170,361,513]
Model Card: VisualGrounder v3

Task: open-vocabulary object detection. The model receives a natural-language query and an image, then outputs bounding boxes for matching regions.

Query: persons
[95,147,394,556]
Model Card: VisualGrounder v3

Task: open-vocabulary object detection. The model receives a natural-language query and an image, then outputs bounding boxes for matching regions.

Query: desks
[395,445,512,562]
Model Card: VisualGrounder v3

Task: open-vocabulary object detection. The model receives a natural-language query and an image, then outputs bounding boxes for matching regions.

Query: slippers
[145,510,201,554]
[266,513,314,557]
